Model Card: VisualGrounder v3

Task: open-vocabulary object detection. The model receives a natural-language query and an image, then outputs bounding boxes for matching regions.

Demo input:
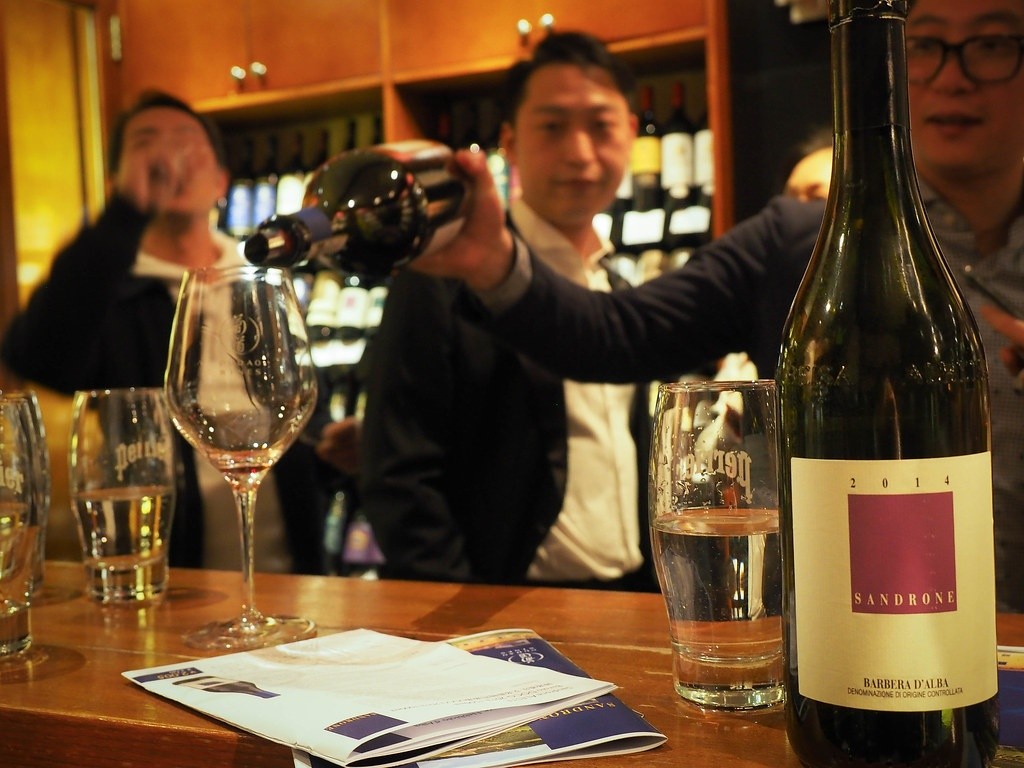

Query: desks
[0,562,1024,768]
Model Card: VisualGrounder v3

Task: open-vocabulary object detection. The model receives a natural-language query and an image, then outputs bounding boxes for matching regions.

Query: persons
[0,87,327,585]
[319,0,1024,611]
[356,28,653,593]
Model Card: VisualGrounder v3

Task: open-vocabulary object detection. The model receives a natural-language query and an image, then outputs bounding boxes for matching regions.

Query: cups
[648,380,787,709]
[68,388,177,605]
[0,390,49,658]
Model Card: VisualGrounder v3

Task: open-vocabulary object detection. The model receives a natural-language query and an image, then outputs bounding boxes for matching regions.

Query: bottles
[245,137,470,291]
[435,79,713,287]
[778,0,998,768]
[225,114,376,239]
[289,271,391,580]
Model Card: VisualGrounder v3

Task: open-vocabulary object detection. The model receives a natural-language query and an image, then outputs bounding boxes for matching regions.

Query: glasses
[903,33,1024,85]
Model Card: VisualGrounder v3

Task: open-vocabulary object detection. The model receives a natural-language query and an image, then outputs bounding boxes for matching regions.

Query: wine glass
[165,265,318,654]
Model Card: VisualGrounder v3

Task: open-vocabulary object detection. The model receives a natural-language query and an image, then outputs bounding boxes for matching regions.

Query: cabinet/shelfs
[113,0,383,119]
[386,0,706,76]
